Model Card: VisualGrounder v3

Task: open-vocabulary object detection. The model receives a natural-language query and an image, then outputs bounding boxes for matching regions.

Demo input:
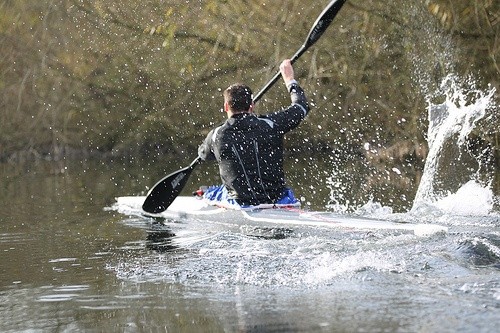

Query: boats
[114,195,500,244]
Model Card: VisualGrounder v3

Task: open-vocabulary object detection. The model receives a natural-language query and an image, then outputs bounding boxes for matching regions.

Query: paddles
[142,0,346,215]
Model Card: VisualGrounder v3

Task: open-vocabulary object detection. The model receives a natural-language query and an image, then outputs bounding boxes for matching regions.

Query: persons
[197,58,310,208]
